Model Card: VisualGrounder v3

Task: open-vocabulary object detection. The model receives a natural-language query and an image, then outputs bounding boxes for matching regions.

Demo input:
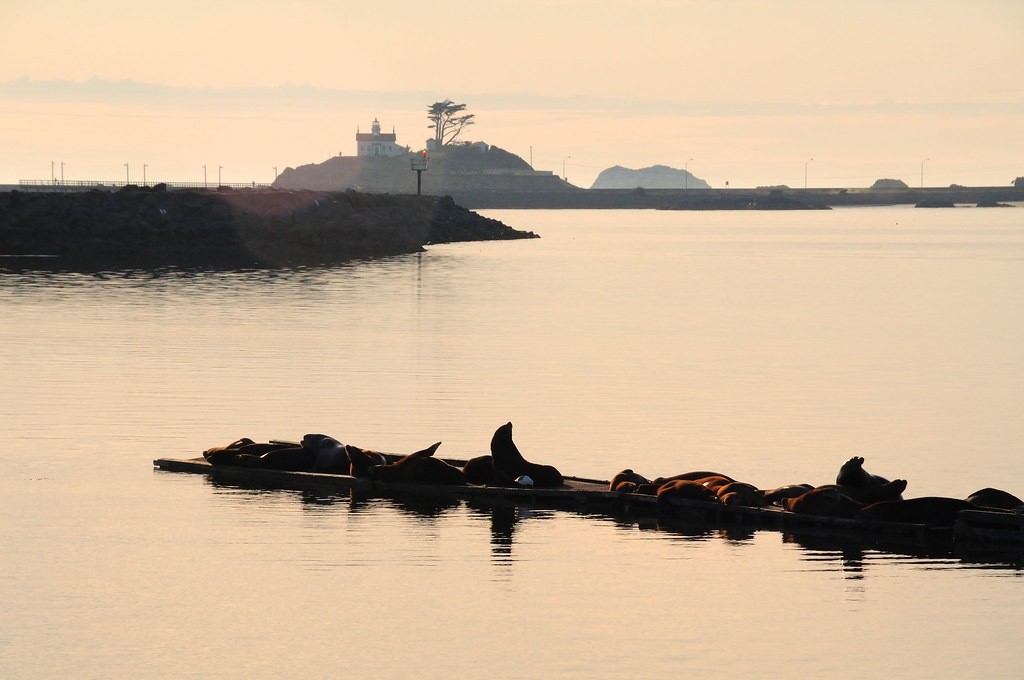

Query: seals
[201,421,1022,561]
